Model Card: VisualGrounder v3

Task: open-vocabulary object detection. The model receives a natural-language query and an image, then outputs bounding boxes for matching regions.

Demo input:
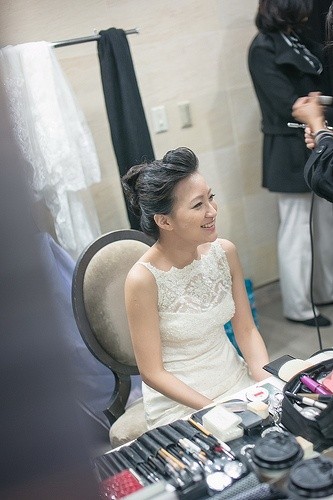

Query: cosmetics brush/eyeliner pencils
[90,418,241,487]
[288,122,333,130]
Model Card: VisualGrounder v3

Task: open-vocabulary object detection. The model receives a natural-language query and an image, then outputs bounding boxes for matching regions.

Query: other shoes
[314,300,332,306]
[284,314,330,327]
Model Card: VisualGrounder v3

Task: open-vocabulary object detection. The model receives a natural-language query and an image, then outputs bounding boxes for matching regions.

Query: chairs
[70,229,158,426]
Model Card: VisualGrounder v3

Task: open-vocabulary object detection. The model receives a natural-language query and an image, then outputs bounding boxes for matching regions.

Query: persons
[291,91,333,204]
[119,147,273,433]
[247,0,333,327]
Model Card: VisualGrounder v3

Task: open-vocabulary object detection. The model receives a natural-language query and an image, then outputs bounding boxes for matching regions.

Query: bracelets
[313,128,333,143]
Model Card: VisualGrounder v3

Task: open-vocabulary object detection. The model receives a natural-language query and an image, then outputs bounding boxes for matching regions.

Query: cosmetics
[205,354,333,500]
